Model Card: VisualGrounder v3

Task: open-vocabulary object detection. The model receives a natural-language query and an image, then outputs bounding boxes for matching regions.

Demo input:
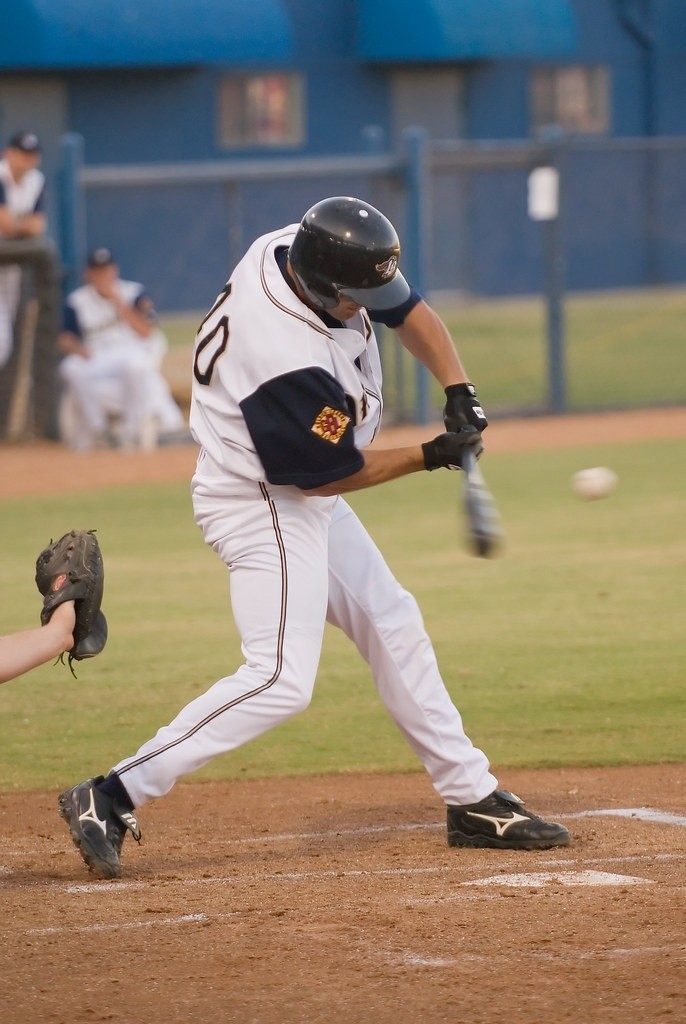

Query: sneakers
[58,775,142,880]
[447,788,570,851]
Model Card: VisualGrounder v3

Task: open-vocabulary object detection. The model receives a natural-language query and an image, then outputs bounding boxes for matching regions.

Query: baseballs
[566,462,625,504]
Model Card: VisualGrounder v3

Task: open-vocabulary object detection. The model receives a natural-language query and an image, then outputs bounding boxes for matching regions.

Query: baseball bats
[453,443,514,566]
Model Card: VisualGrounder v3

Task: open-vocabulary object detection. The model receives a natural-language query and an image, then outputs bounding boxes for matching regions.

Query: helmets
[87,244,117,269]
[290,195,410,311]
[8,130,43,154]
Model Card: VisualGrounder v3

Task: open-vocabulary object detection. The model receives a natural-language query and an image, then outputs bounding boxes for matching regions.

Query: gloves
[422,422,484,471]
[443,383,487,434]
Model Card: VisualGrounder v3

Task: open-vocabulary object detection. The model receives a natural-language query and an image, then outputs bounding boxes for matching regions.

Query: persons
[57,196,570,878]
[0,132,187,451]
[0,530,108,684]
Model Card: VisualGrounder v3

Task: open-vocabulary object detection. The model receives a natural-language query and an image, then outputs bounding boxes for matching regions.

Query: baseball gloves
[28,525,112,668]
